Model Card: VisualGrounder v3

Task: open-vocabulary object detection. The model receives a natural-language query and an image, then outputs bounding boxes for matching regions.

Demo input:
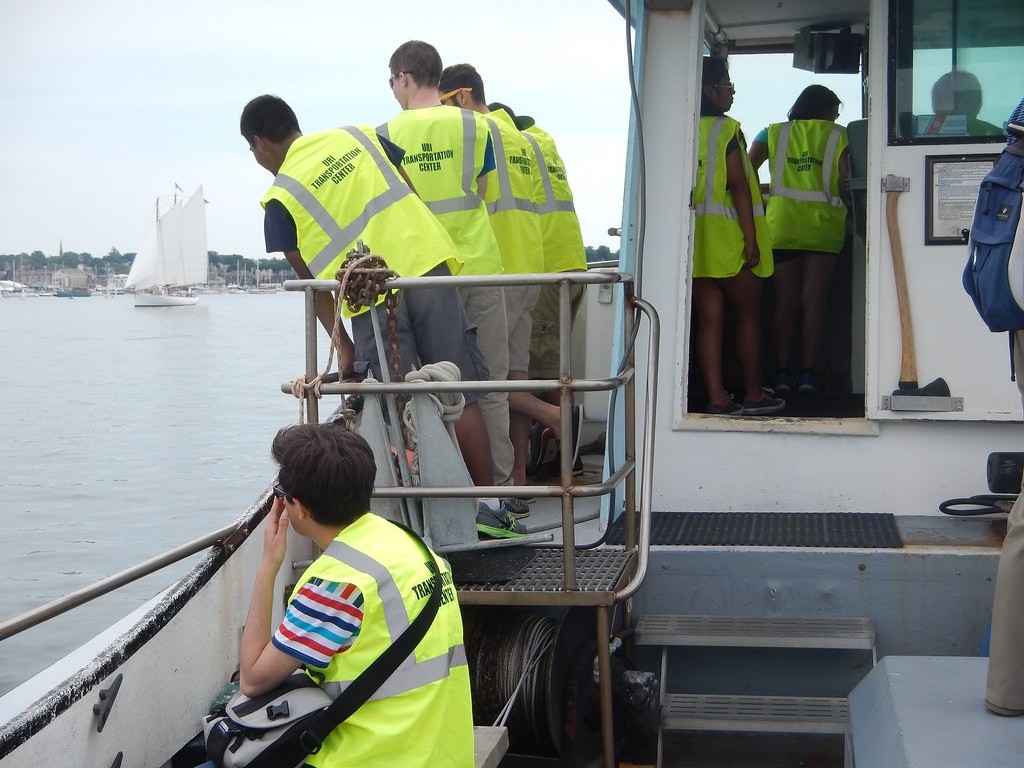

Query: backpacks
[962,95,1024,333]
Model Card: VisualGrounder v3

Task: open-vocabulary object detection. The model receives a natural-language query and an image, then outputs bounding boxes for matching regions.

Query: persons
[748,85,849,396]
[692,57,786,416]
[240,40,588,539]
[912,70,1003,136]
[986,100,1024,718]
[192,423,477,768]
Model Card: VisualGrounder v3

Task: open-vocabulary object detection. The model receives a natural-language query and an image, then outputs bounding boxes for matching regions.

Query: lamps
[793,26,863,74]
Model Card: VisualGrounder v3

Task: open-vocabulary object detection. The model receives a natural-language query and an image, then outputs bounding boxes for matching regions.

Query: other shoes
[799,368,816,393]
[775,368,792,394]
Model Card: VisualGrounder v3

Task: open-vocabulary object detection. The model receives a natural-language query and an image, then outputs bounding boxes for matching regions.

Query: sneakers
[708,391,744,415]
[499,498,529,518]
[476,500,528,538]
[743,387,785,414]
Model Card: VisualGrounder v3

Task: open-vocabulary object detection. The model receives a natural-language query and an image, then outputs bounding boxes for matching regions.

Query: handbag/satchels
[202,666,334,768]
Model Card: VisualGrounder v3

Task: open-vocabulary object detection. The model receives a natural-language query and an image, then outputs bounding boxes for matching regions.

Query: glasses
[273,483,294,500]
[711,83,734,93]
[439,88,472,105]
[389,72,414,90]
[831,110,840,119]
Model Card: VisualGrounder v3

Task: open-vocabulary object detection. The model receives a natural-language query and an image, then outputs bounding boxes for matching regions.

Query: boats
[102,288,126,294]
[39,291,52,297]
[248,286,276,294]
[52,291,91,297]
[1,290,40,297]
[226,283,246,294]
[277,286,285,292]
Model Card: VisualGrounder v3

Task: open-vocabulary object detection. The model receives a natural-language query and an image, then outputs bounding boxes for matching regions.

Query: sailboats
[124,182,210,307]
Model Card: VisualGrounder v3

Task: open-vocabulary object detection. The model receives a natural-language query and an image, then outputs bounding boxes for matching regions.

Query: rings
[755,259,758,262]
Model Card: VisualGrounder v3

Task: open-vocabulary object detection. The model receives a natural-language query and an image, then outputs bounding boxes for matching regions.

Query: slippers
[555,405,584,470]
